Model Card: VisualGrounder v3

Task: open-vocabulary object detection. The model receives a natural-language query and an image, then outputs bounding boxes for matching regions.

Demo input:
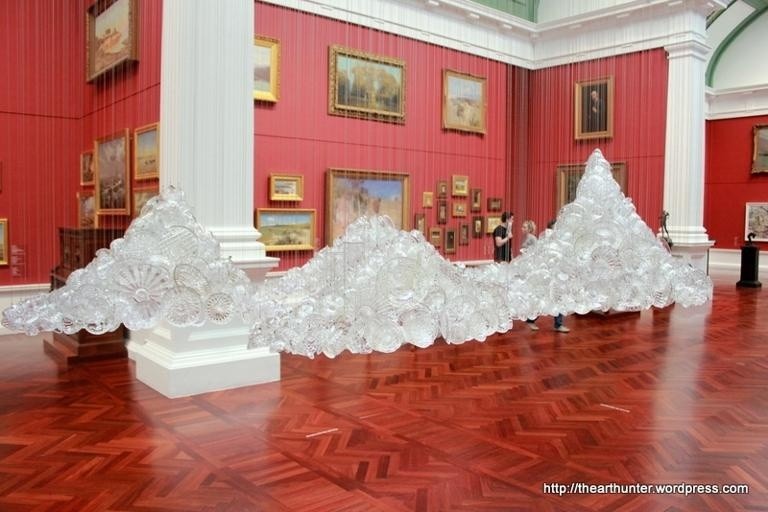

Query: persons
[518,219,539,253]
[524,219,571,333]
[584,88,606,131]
[492,210,515,262]
[439,184,446,197]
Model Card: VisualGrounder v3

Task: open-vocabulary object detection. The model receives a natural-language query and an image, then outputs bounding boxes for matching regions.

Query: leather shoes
[553,325,570,332]
[527,323,539,331]
